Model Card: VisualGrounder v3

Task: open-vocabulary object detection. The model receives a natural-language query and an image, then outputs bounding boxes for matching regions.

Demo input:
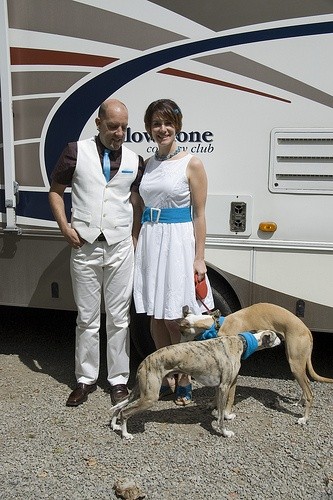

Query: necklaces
[155,149,180,161]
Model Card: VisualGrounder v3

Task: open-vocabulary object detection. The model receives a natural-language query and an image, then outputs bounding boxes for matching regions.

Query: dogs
[107,327,283,440]
[171,301,333,426]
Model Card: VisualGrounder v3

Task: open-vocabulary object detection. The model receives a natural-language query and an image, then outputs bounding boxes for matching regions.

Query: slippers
[158,384,176,400]
[174,381,193,405]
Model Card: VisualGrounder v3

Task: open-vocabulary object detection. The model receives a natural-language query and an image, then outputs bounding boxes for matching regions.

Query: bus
[0,1,333,378]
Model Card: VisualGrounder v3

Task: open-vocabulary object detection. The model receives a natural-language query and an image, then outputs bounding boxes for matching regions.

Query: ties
[103,149,111,183]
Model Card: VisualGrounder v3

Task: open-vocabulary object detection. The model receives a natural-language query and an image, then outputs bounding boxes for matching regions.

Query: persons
[133,99,215,405]
[48,99,144,407]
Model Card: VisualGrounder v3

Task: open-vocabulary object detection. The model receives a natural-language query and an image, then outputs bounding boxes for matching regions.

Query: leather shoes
[66,382,97,407]
[110,384,130,406]
